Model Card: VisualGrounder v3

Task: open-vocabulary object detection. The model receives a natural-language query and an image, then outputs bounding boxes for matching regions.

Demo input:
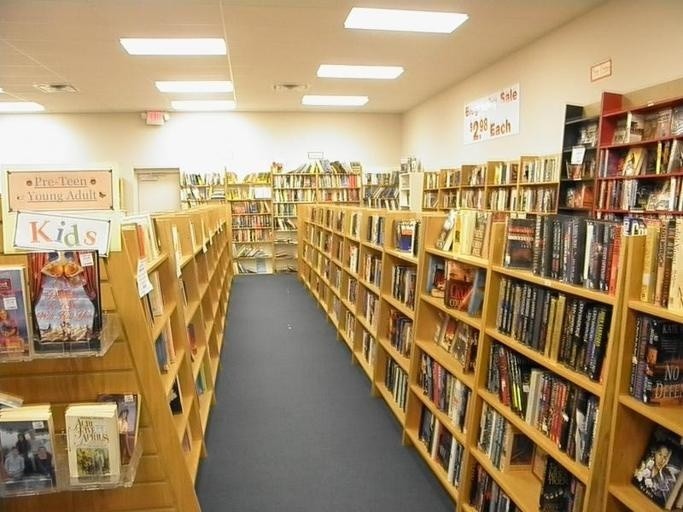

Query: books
[0,392,57,490]
[64,390,141,486]
[183,159,361,274]
[120,213,204,416]
[307,107,683,511]
[1,250,102,357]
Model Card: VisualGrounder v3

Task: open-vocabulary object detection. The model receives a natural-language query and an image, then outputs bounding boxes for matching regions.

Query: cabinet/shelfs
[365,171,398,210]
[398,171,424,211]
[424,153,560,215]
[179,165,364,275]
[557,79,681,217]
[297,200,682,512]
[1,203,238,512]
